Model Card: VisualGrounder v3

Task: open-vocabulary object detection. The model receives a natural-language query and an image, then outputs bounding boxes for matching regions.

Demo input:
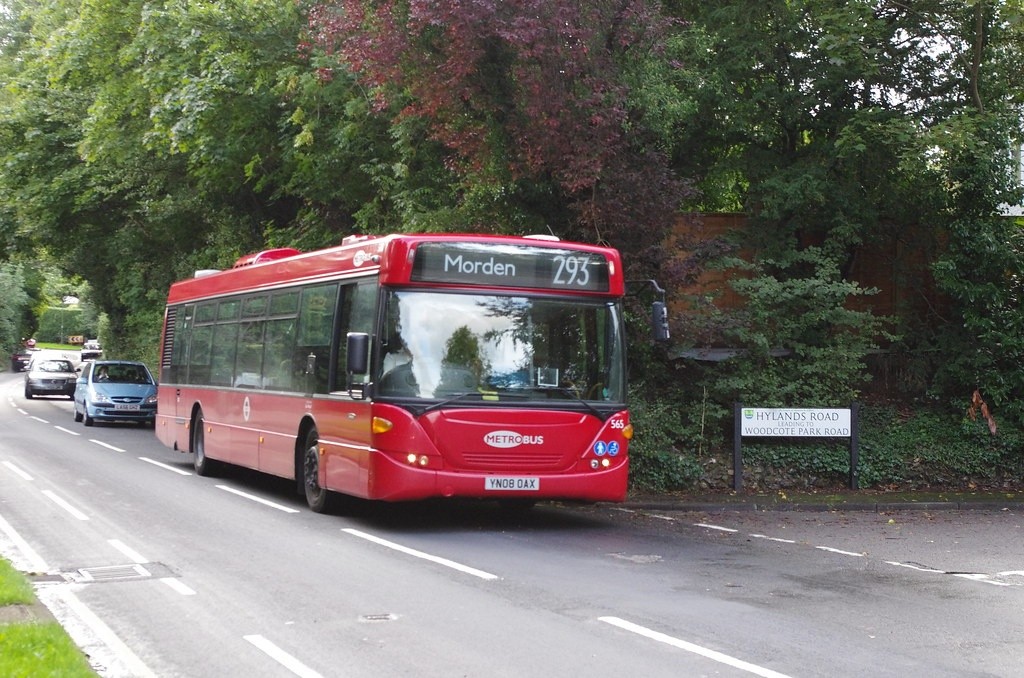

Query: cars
[73,360,158,430]
[11,340,103,402]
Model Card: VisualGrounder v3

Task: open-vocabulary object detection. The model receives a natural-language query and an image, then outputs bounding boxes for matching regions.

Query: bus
[154,232,671,517]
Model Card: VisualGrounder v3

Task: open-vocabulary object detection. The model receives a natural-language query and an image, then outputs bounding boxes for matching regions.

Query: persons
[96,365,112,382]
[383,331,413,375]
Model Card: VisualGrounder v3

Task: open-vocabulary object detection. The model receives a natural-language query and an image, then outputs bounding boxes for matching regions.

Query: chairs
[188,343,293,390]
